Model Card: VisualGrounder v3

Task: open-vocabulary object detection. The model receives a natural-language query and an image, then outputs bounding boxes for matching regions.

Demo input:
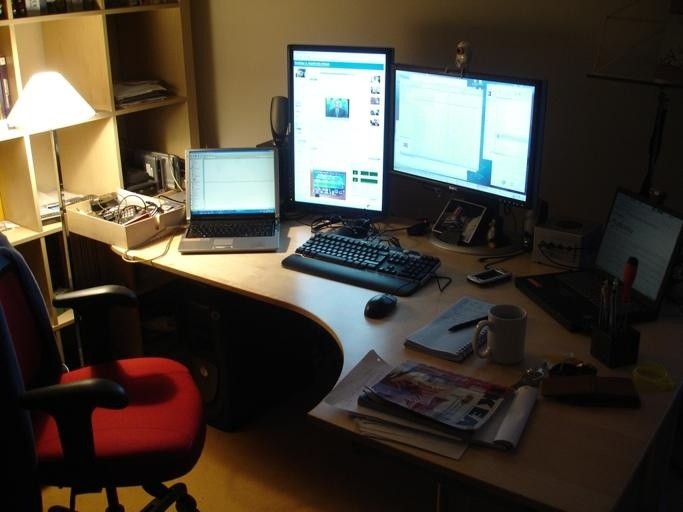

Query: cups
[472,304,528,365]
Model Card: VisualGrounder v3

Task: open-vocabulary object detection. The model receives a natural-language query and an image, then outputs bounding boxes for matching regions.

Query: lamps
[3,69,98,372]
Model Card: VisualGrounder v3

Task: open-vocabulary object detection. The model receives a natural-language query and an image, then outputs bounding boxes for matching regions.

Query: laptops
[177,146,282,255]
[514,186,683,333]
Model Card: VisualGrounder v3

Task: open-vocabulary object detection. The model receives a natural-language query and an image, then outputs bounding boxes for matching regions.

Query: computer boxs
[174,282,292,431]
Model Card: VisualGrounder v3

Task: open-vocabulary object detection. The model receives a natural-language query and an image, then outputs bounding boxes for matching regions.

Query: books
[405,295,498,362]
[358,359,515,442]
[0,56,12,120]
[11,0,84,16]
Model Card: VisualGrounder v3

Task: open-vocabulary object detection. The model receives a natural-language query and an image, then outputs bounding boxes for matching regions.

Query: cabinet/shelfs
[0,1,201,375]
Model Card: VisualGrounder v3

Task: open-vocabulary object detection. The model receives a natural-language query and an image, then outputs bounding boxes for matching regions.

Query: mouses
[364,292,398,319]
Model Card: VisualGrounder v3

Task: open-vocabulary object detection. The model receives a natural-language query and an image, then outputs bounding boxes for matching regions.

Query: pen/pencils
[598,277,621,333]
[448,315,488,332]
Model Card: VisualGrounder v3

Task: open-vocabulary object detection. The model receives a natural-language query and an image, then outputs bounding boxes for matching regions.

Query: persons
[329,100,347,117]
[440,206,465,238]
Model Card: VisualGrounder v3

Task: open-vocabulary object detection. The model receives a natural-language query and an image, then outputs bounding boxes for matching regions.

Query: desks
[107,195,681,511]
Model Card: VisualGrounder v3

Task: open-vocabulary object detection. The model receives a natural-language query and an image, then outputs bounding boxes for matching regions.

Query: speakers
[270,95,288,146]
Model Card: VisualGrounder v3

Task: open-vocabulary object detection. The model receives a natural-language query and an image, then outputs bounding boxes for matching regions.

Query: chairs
[1,232,204,512]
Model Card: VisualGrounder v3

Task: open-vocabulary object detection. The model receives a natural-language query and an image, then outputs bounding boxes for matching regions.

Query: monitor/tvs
[284,41,396,221]
[389,62,549,257]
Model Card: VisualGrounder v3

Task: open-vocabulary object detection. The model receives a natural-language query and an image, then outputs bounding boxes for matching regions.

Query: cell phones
[467,266,512,285]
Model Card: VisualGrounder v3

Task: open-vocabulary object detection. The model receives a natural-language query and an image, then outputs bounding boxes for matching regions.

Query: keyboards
[281,230,443,297]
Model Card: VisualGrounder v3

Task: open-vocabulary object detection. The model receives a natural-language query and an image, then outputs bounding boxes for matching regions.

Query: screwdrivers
[618,255,640,331]
